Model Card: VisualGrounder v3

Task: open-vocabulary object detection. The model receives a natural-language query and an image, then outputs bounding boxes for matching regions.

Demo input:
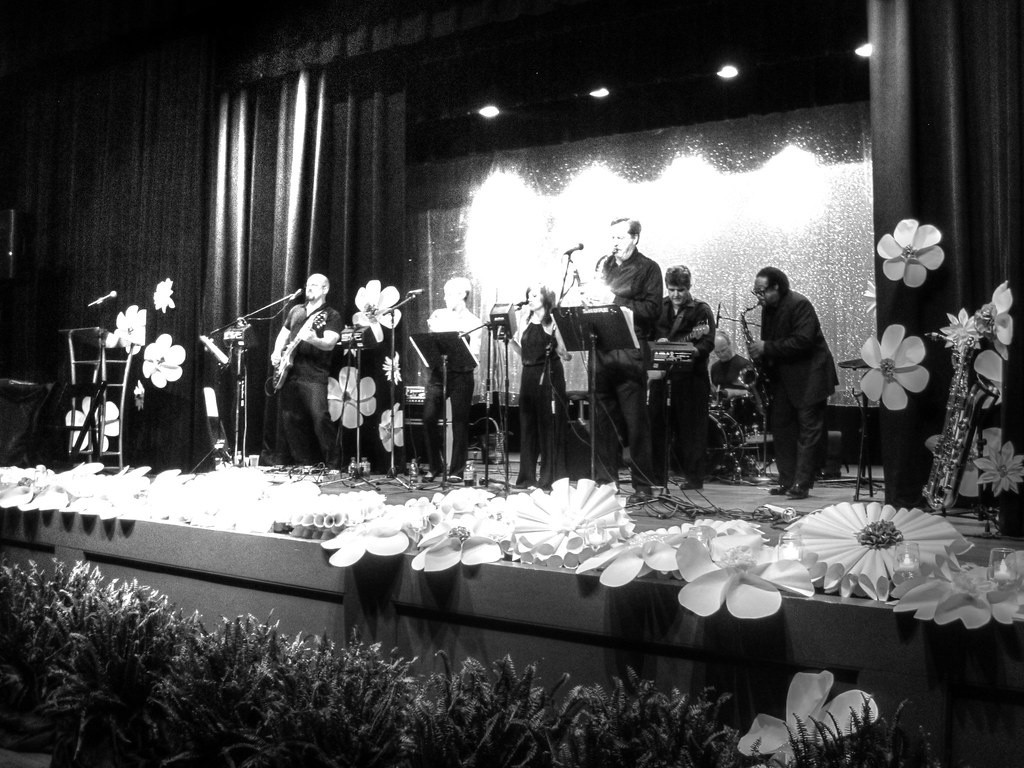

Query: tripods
[623,363,696,521]
[318,294,479,497]
[816,389,886,489]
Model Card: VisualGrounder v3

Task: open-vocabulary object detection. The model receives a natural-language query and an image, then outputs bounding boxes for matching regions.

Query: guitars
[645,319,711,382]
[272,313,330,390]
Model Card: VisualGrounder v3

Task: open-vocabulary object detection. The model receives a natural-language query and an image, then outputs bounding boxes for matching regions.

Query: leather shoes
[770,484,787,495]
[786,483,810,500]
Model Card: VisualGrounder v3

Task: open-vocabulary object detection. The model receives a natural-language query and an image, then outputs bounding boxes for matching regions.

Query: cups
[248,455,260,466]
[686,525,718,553]
[777,532,804,562]
[0,467,12,486]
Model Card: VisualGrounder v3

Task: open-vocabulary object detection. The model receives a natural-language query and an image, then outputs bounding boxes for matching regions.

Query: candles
[993,559,1011,580]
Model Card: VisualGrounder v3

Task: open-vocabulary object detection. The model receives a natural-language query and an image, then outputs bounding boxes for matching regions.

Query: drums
[710,409,746,480]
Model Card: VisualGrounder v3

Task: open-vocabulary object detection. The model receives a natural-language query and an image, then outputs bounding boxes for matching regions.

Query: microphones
[565,243,584,255]
[288,288,304,302]
[715,304,721,328]
[88,291,118,308]
[408,289,426,294]
[517,301,530,306]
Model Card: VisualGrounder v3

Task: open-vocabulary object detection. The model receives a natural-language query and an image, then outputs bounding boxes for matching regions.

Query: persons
[269,273,346,470]
[663,265,716,493]
[504,281,573,493]
[750,267,840,500]
[586,215,664,508]
[711,328,758,443]
[417,277,483,483]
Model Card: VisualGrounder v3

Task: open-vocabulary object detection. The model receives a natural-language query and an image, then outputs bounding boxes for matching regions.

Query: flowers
[0,218,1024,631]
[736,670,879,768]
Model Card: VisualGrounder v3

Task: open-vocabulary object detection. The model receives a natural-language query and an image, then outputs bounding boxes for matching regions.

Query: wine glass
[583,518,609,572]
[34,470,48,492]
[408,504,429,553]
[986,547,1017,607]
[893,541,921,582]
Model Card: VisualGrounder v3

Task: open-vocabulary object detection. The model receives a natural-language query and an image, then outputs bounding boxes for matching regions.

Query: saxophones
[736,299,779,419]
[923,332,1001,510]
[588,250,618,303]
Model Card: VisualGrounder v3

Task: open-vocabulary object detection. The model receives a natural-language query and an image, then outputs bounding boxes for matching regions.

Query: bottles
[408,458,418,486]
[463,460,477,486]
[348,456,370,479]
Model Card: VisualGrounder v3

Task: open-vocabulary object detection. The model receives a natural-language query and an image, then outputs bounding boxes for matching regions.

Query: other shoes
[678,483,703,490]
[447,473,464,483]
[421,464,444,483]
[629,492,650,503]
